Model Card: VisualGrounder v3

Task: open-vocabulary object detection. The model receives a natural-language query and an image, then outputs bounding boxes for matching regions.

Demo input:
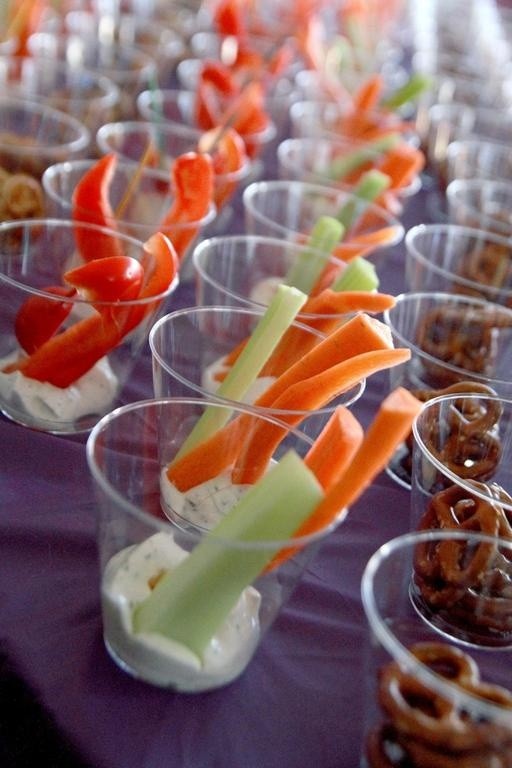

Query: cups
[1,0,512,766]
